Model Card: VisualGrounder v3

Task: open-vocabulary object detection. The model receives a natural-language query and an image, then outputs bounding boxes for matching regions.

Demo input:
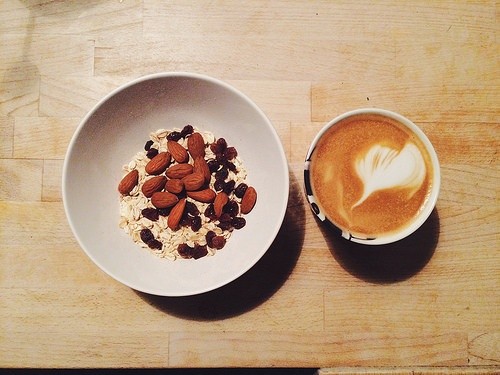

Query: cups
[304,108,441,246]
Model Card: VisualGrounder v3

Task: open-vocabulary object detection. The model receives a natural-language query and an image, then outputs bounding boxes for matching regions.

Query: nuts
[118,125,256,230]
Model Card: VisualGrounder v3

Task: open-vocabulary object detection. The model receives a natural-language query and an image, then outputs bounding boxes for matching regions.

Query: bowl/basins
[61,72,289,297]
